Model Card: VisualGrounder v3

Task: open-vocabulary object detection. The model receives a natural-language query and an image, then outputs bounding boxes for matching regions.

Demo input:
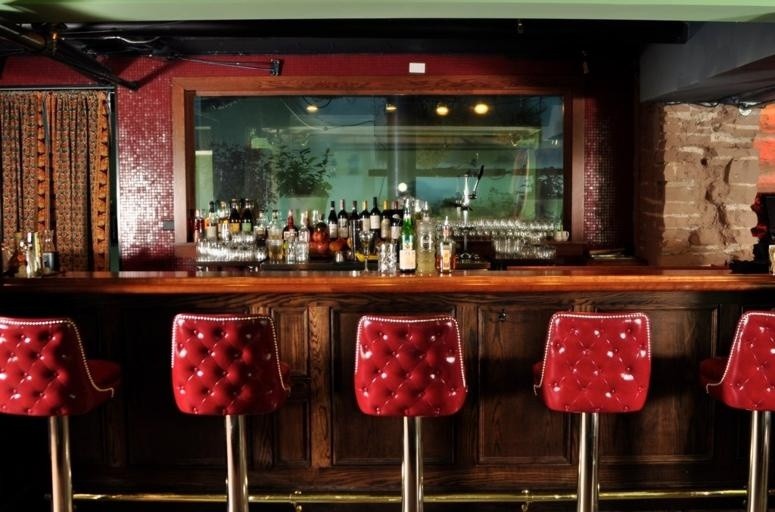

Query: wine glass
[358,231,375,274]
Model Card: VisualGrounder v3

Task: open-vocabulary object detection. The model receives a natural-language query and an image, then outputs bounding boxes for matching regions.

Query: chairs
[0,316,116,512]
[172,313,291,512]
[353,314,468,512]
[702,312,774,511]
[535,309,651,512]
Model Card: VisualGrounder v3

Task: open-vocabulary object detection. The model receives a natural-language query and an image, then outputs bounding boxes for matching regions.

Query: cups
[42,251,59,275]
[458,218,561,260]
[194,230,309,262]
[377,243,398,277]
[553,230,570,242]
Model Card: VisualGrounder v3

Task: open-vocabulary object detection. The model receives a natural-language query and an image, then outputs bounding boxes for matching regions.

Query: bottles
[193,198,254,242]
[254,208,314,250]
[13,229,55,278]
[314,196,398,256]
[398,199,458,275]
